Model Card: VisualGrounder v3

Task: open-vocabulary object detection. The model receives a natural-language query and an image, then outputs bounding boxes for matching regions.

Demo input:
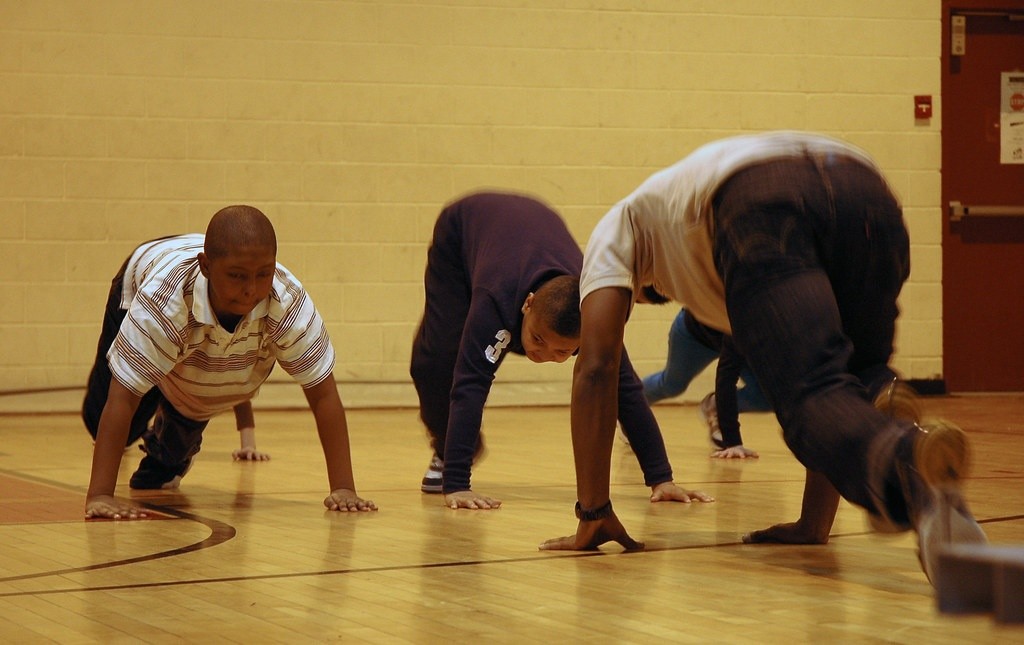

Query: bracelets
[574,499,612,521]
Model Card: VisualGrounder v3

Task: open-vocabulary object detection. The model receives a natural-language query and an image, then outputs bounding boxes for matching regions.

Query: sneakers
[421,431,484,491]
[701,392,724,447]
[129,429,196,489]
[617,419,630,445]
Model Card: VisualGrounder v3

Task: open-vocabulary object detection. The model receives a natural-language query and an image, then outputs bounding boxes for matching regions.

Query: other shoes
[894,426,994,609]
[867,380,923,539]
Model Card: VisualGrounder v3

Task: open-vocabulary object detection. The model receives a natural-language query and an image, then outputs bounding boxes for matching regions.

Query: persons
[82,202,379,522]
[411,194,718,512]
[620,300,773,459]
[538,131,997,608]
